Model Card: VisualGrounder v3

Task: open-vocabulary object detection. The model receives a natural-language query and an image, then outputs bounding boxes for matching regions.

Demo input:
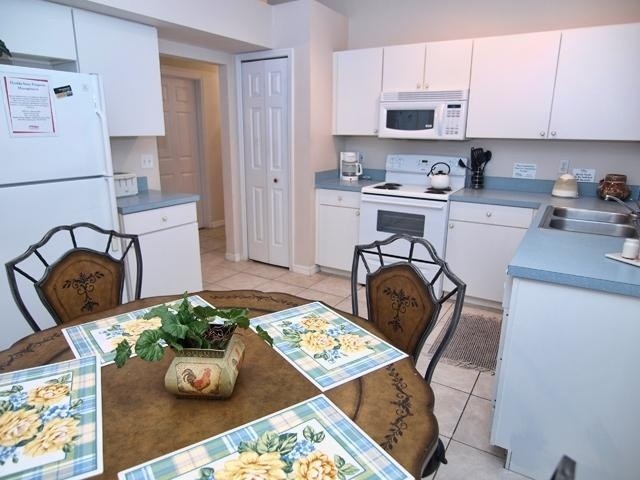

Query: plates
[604,252,640,267]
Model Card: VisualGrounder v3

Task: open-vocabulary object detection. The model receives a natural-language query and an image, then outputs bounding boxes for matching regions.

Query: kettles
[552,173,580,199]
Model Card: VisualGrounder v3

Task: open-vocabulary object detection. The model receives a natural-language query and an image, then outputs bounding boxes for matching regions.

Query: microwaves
[377,100,468,141]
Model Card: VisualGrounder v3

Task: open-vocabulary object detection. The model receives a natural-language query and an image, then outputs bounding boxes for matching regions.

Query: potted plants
[113,291,276,399]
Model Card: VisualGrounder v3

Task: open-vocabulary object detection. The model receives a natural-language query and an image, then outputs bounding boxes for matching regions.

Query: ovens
[356,193,447,299]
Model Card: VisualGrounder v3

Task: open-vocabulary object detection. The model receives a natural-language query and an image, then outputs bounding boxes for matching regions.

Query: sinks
[539,215,639,242]
[538,205,635,229]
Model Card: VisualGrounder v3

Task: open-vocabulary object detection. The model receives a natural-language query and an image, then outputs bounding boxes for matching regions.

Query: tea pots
[426,162,451,191]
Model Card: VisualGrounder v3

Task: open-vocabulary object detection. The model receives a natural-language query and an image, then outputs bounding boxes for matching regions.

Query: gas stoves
[362,154,469,202]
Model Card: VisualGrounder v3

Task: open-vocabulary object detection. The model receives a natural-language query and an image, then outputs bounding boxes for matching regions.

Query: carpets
[427,309,503,377]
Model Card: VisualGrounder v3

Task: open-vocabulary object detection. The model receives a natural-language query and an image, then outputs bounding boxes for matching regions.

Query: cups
[621,237,640,258]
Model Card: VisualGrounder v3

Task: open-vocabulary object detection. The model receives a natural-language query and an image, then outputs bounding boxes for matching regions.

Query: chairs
[4,221,145,334]
[349,233,467,478]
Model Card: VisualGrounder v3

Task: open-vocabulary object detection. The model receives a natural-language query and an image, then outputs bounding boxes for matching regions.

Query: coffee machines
[339,151,363,182]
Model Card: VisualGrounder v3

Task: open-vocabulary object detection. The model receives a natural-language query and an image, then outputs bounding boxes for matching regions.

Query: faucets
[606,195,639,221]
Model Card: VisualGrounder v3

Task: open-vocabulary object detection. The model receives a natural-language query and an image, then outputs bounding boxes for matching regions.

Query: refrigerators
[1,62,134,351]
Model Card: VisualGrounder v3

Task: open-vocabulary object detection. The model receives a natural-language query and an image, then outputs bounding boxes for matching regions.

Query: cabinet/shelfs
[466,23,639,142]
[313,189,362,278]
[332,47,383,137]
[115,190,204,306]
[442,200,539,314]
[2,1,165,138]
[378,38,472,141]
